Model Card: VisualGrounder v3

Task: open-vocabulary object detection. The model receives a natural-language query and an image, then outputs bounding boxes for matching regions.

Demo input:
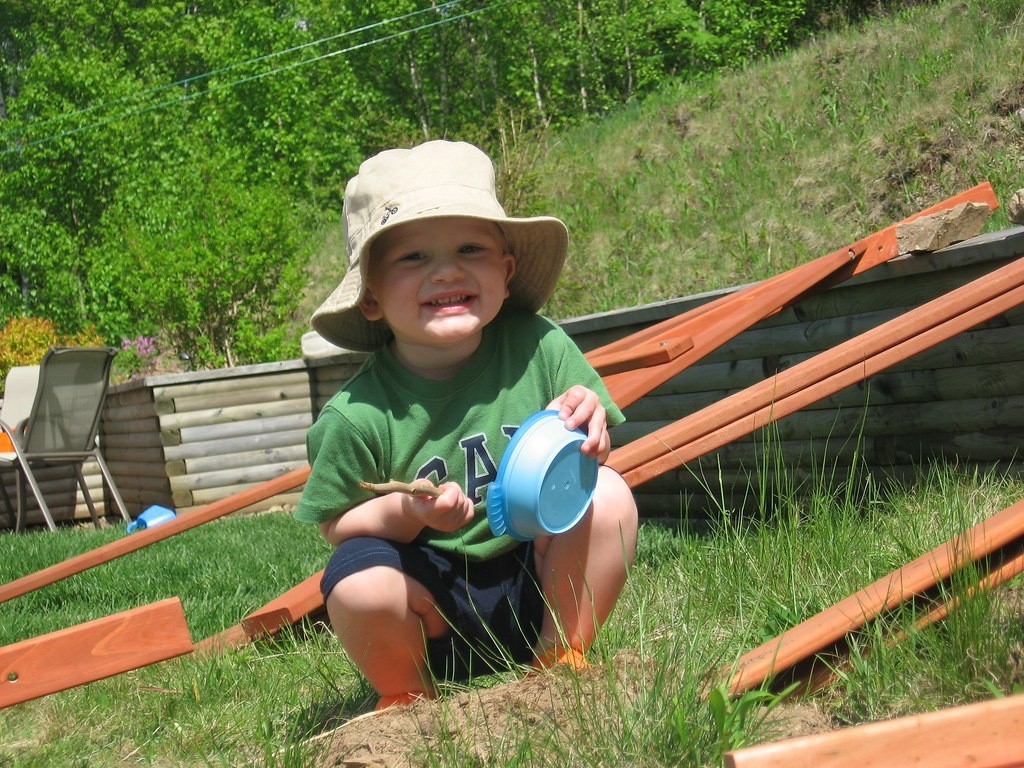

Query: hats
[308,139,569,353]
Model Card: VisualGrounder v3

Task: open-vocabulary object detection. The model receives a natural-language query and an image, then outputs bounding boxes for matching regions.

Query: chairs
[0,346,133,536]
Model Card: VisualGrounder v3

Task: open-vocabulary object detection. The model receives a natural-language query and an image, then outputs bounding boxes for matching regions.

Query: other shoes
[530,645,589,677]
[372,689,432,711]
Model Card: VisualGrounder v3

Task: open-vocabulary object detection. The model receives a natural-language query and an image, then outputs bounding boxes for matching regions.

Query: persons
[293,140,638,713]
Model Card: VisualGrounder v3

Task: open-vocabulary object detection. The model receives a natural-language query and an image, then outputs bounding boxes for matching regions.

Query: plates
[485,410,598,541]
